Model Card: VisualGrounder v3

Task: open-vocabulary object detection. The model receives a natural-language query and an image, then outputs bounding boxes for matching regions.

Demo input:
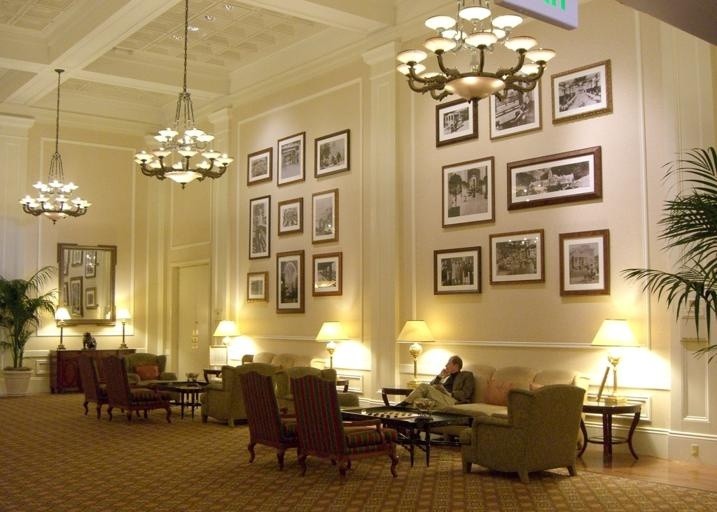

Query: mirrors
[58,242,116,325]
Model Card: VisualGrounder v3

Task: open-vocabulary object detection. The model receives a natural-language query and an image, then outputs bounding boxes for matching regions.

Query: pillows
[135,361,160,379]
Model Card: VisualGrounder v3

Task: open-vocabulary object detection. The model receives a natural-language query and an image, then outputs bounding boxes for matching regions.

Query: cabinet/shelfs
[49,349,136,394]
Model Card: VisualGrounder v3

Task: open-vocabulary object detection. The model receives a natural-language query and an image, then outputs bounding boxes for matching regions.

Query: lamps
[590,320,640,404]
[19,69,93,225]
[315,321,352,369]
[134,0,235,190]
[213,320,240,365]
[397,0,558,103]
[55,307,72,350]
[396,320,438,388]
[116,309,132,348]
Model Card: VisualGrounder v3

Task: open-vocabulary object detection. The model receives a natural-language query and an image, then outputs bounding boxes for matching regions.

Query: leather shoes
[395,401,413,409]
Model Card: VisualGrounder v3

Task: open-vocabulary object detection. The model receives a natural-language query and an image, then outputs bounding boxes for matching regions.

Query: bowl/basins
[413,399,439,411]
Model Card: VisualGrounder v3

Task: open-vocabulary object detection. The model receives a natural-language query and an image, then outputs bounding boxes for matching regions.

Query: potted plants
[0,265,63,397]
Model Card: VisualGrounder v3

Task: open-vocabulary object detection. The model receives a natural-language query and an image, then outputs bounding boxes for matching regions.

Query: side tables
[577,401,641,469]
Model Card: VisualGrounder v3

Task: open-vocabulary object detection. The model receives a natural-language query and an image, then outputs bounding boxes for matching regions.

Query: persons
[397,356,475,439]
[279,261,299,302]
[253,205,267,254]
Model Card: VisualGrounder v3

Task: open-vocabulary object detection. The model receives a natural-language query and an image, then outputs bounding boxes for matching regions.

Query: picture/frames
[442,156,495,228]
[72,249,83,266]
[278,197,303,236]
[64,249,69,264]
[559,229,610,296]
[247,271,269,303]
[507,146,601,210]
[69,276,83,318]
[436,97,478,147]
[247,147,273,186]
[434,246,481,295]
[85,287,96,309]
[311,188,339,244]
[64,282,69,306]
[84,250,97,278]
[489,77,542,141]
[249,195,271,260]
[595,366,610,402]
[276,250,305,313]
[312,252,342,296]
[64,264,68,275]
[314,129,350,178]
[489,230,545,284]
[277,132,305,186]
[551,59,613,125]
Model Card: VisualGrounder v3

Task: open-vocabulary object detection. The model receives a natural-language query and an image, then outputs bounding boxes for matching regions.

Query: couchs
[274,367,359,416]
[459,384,586,484]
[200,363,283,427]
[204,352,327,385]
[123,353,180,405]
[382,363,591,450]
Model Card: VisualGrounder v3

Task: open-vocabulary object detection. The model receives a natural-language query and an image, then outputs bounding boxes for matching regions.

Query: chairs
[239,371,298,471]
[289,375,399,485]
[78,352,142,419]
[101,355,172,423]
